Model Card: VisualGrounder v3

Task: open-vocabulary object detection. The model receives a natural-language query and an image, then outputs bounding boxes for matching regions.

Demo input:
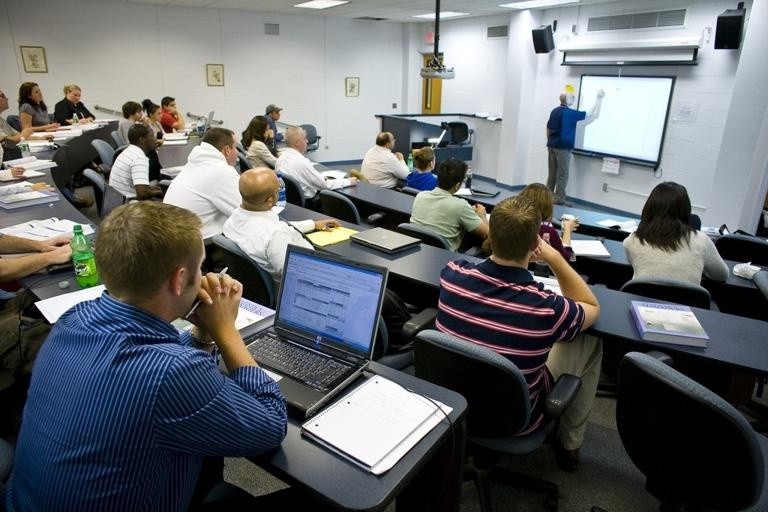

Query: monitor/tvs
[719,223,730,235]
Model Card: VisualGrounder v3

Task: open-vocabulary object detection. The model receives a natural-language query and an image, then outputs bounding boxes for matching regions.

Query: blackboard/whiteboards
[571,74,677,168]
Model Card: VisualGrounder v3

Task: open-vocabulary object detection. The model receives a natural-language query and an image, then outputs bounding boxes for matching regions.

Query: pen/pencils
[6,165,13,168]
[24,184,54,187]
[186,267,228,318]
[322,224,332,231]
[575,216,582,220]
[80,112,85,119]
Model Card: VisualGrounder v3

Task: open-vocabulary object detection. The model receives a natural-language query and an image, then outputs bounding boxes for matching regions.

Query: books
[595,218,639,233]
[1,180,61,211]
[629,299,710,349]
[300,374,445,476]
[567,239,611,258]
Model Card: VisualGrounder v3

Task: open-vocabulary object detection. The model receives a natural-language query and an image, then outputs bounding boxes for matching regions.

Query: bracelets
[190,337,215,349]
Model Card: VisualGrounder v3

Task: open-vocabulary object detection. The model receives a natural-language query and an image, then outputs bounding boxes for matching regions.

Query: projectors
[420,66,454,80]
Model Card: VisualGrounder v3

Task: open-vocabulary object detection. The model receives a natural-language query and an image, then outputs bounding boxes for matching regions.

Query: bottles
[72,113,80,132]
[407,153,414,173]
[19,136,30,158]
[276,173,287,207]
[70,224,98,287]
[465,165,472,188]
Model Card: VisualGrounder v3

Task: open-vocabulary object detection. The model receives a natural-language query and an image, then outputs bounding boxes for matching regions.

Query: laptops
[349,226,422,255]
[215,242,391,420]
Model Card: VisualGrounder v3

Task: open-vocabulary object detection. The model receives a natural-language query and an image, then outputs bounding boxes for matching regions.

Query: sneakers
[76,195,93,207]
[564,200,572,207]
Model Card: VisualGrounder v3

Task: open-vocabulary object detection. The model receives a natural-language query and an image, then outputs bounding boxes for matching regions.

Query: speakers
[714,9,746,50]
[532,23,555,54]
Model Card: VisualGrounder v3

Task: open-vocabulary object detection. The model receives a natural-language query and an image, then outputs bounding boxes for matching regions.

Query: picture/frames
[345,77,360,98]
[19,46,49,74]
[206,64,225,87]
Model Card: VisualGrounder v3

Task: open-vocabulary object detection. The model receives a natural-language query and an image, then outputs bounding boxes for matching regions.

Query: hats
[266,104,283,113]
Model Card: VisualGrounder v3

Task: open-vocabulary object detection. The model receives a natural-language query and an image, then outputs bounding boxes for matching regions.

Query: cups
[559,213,576,240]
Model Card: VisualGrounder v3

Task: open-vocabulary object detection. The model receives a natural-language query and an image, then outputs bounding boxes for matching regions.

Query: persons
[406,146,439,191]
[0,80,96,170]
[109,97,186,204]
[518,183,580,264]
[435,195,605,470]
[409,156,490,253]
[358,131,409,191]
[0,232,73,393]
[0,199,289,511]
[621,180,729,288]
[161,103,361,275]
[545,87,605,208]
